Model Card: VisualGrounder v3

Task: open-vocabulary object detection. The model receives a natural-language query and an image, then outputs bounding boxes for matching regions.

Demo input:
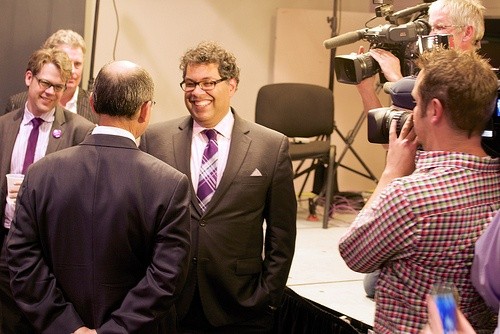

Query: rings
[379,54,383,58]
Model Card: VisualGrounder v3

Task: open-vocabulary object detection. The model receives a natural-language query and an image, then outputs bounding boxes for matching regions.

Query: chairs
[255,83,335,228]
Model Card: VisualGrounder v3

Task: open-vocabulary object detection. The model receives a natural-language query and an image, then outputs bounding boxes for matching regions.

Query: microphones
[324,29,366,49]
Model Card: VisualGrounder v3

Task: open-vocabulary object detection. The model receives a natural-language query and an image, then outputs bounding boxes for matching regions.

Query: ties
[22,117,45,175]
[197,128,219,213]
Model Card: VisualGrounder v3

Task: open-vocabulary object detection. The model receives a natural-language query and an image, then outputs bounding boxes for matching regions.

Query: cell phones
[432,282,458,334]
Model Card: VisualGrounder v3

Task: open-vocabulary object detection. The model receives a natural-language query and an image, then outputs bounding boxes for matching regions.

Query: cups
[6,173,25,203]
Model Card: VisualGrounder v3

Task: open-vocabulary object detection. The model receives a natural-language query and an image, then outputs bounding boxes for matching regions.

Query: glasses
[180,76,229,93]
[429,23,466,34]
[30,71,68,92]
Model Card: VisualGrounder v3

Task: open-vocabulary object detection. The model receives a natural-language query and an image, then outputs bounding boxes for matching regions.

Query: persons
[7,29,99,127]
[0,47,96,334]
[5,60,193,334]
[419,211,500,334]
[339,48,500,334]
[349,0,500,160]
[135,42,298,334]
